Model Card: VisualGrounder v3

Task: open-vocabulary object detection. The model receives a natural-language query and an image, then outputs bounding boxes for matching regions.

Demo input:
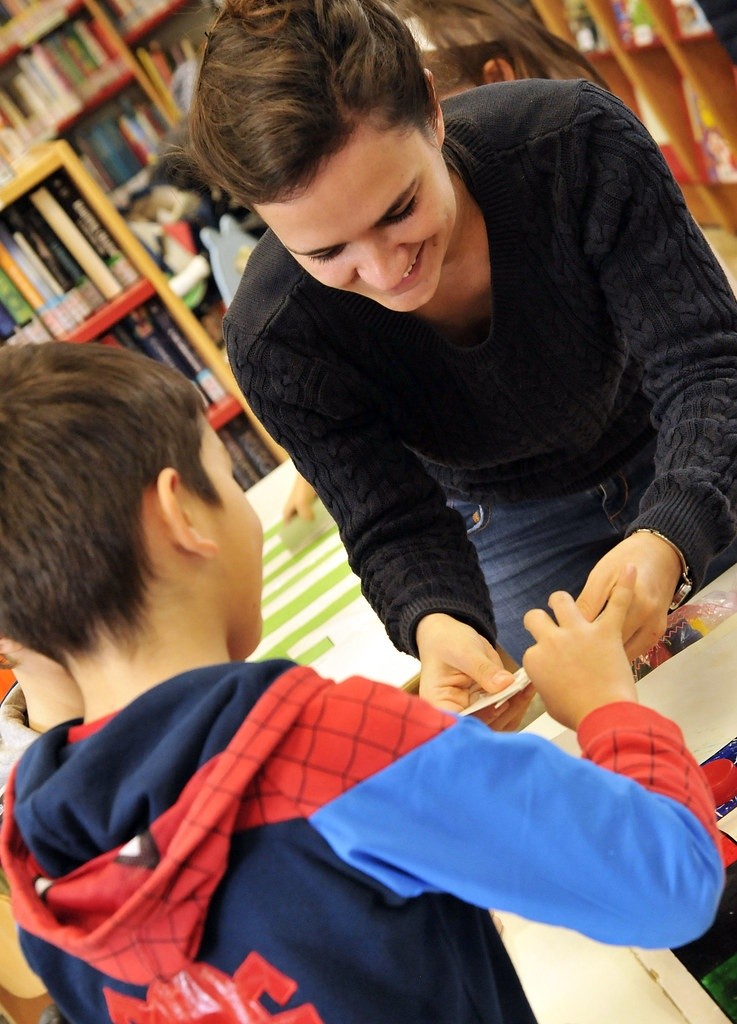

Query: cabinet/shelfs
[2,0,737,530]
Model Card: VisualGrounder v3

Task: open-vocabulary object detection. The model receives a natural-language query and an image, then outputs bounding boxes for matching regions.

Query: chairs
[244,494,422,688]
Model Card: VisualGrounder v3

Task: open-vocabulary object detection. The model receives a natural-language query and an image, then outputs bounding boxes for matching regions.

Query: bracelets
[631,529,692,610]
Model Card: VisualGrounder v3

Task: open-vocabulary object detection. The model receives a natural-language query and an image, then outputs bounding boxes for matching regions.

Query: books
[2,0,289,492]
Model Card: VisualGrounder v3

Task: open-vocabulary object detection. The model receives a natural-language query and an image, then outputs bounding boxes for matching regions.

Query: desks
[488,561,737,1024]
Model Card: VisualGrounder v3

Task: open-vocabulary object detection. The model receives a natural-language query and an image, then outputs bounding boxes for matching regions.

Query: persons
[183,0,737,734]
[0,634,85,1024]
[0,338,728,1024]
